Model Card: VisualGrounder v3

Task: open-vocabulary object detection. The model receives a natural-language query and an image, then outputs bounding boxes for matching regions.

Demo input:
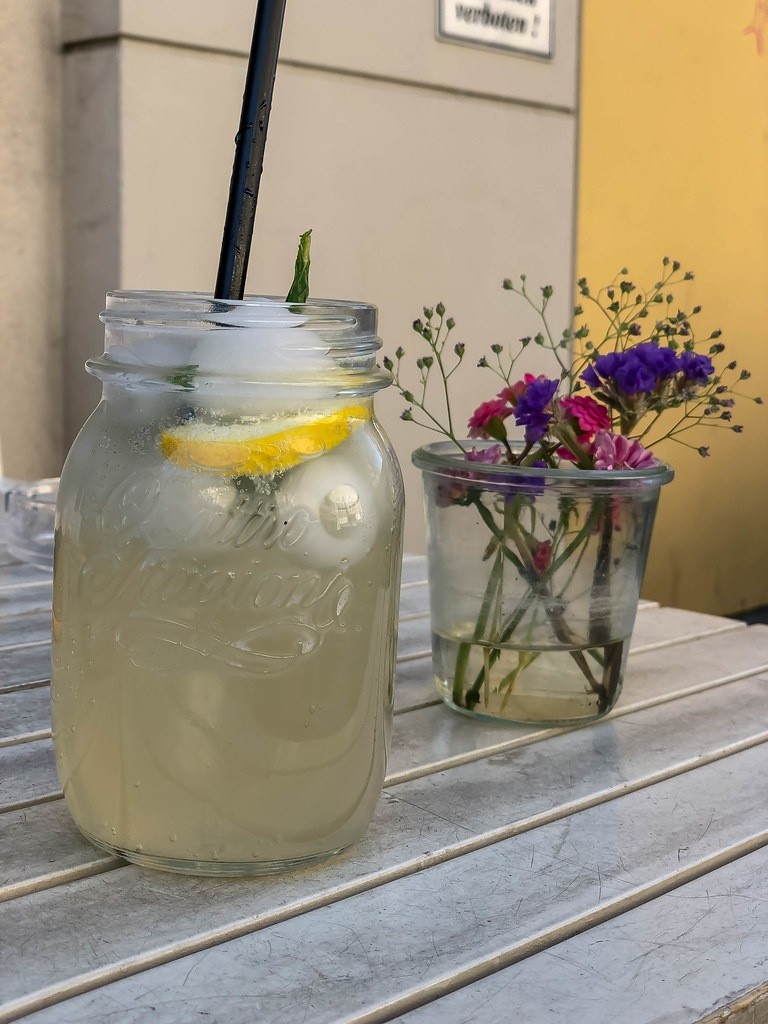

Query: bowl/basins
[5,477,61,573]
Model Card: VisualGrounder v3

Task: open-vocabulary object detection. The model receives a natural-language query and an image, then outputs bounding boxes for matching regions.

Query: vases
[411,436,674,730]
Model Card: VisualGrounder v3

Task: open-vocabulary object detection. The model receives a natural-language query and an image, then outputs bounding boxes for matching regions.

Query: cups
[54,290,405,886]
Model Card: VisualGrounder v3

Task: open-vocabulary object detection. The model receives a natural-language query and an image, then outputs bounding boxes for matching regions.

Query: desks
[0,549,768,1024]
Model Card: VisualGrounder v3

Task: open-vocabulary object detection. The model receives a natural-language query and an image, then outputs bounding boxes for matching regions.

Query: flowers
[376,256,763,732]
